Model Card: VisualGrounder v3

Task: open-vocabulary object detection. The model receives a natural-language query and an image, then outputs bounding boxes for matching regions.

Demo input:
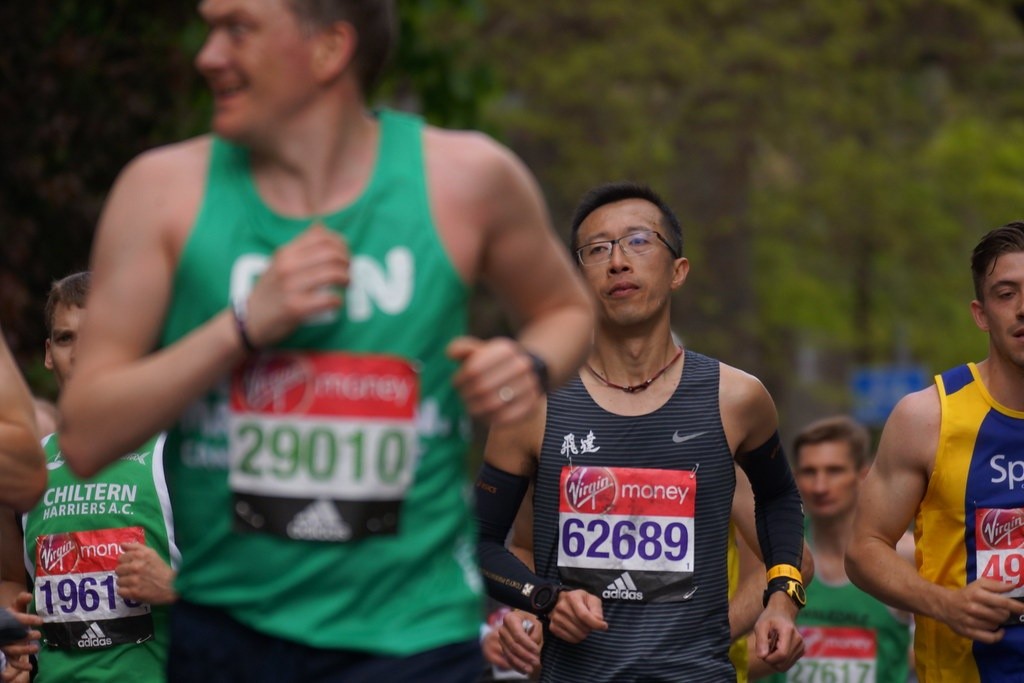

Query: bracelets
[522,349,548,396]
[234,299,261,355]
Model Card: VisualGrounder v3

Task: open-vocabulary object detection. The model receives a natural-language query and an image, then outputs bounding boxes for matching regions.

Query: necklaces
[584,346,683,393]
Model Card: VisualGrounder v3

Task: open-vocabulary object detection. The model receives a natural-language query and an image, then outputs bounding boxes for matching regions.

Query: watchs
[529,582,574,625]
[763,582,807,608]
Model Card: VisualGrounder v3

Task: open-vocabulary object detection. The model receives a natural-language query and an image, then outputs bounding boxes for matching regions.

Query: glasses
[575,230,681,268]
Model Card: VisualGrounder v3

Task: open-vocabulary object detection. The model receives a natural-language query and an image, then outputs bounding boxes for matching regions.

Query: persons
[0,268,177,683]
[747,417,918,683]
[55,0,598,683]
[844,222,1024,683]
[470,186,815,683]
